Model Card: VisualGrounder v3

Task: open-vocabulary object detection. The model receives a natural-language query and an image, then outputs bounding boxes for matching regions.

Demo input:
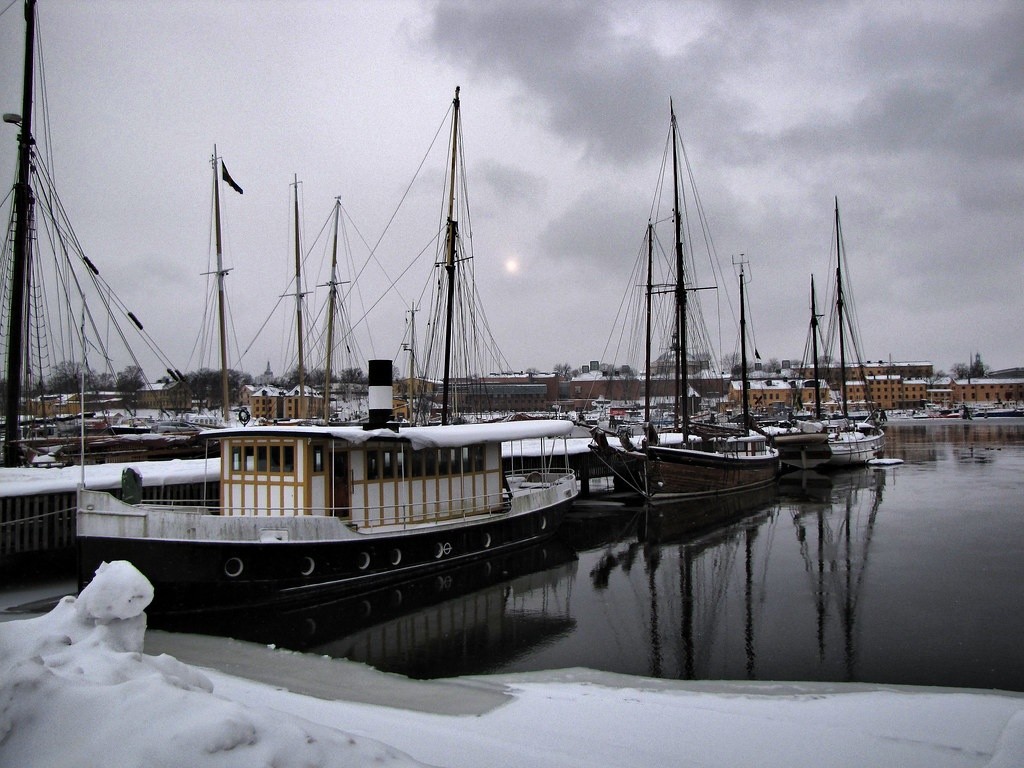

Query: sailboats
[568,95,783,502]
[72,82,583,614]
[767,193,886,470]
[0,0,229,470]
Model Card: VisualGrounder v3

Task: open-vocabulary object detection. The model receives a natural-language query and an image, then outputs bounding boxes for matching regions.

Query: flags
[222,162,243,195]
